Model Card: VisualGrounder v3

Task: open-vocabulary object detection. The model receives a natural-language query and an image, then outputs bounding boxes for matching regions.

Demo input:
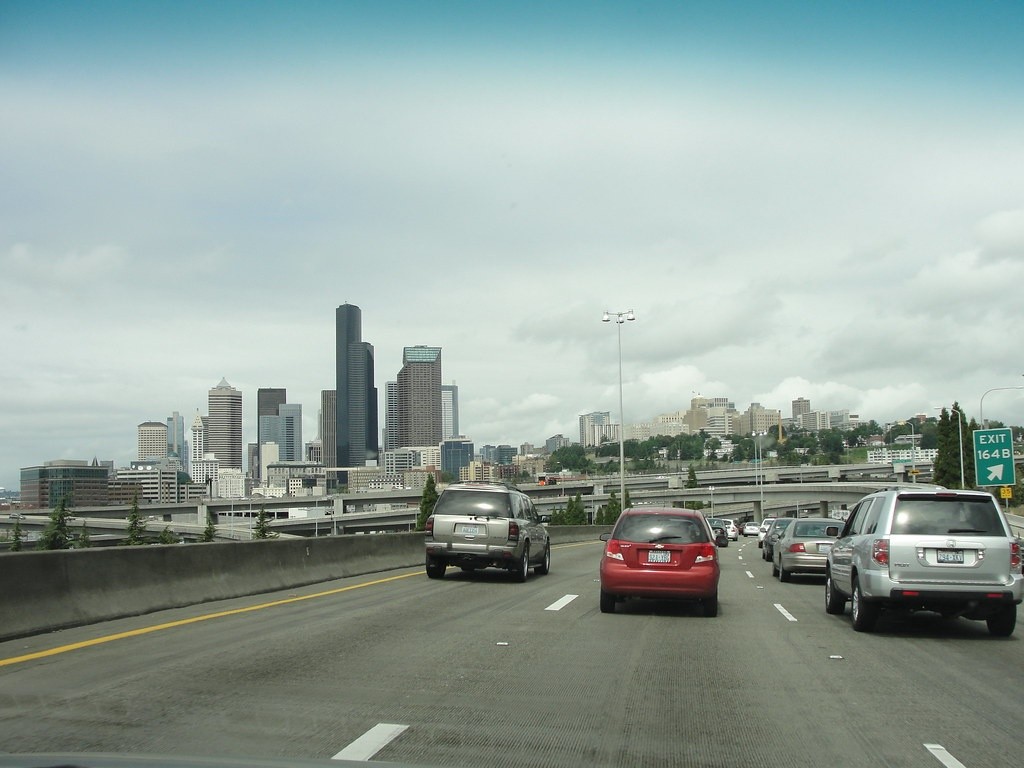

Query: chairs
[679,522,696,538]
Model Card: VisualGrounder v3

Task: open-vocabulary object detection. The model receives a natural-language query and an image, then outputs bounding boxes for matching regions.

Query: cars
[760,517,806,563]
[771,517,847,583]
[600,507,729,618]
[693,517,728,547]
[757,518,788,548]
[740,522,747,536]
[743,521,761,537]
[714,518,739,541]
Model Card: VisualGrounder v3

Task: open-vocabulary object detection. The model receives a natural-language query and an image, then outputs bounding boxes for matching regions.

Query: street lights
[896,421,916,483]
[603,308,636,512]
[934,407,964,490]
[752,429,768,521]
[557,461,565,497]
[979,387,1024,432]
[709,485,715,519]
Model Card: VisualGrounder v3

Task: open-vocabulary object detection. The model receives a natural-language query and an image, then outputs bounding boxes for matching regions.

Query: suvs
[824,490,1024,639]
[425,482,552,583]
